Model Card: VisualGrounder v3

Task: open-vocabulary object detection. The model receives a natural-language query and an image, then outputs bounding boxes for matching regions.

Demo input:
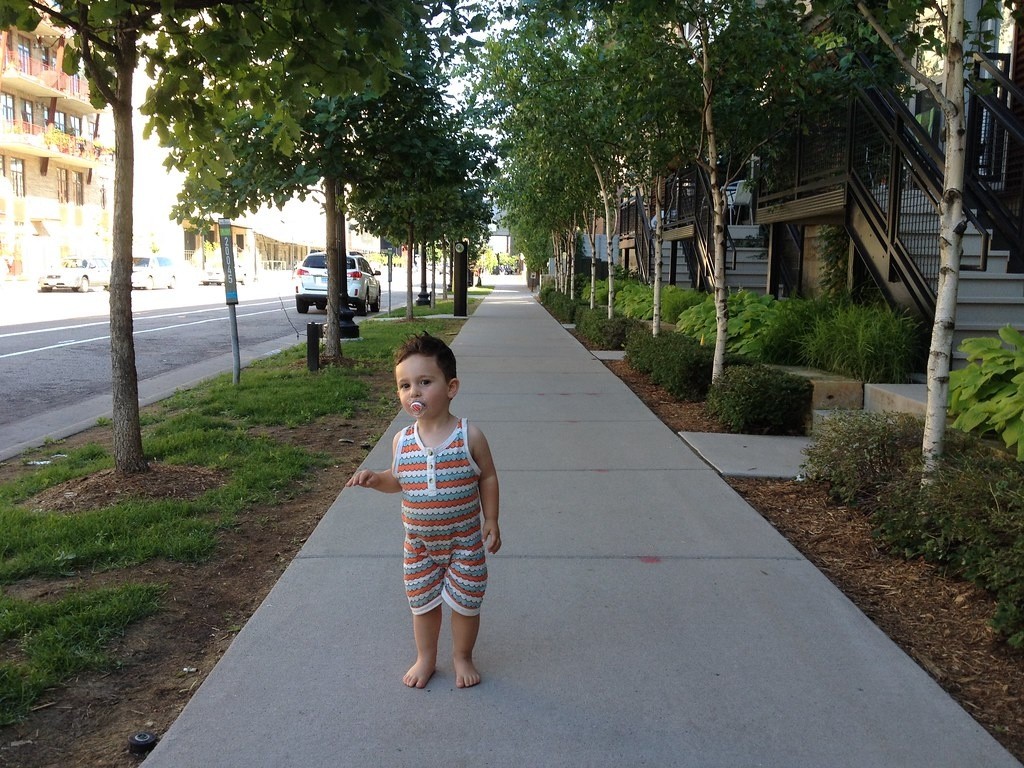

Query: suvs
[294,250,382,316]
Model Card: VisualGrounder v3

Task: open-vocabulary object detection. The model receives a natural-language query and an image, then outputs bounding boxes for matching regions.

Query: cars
[199,261,249,285]
[129,253,179,291]
[37,256,112,292]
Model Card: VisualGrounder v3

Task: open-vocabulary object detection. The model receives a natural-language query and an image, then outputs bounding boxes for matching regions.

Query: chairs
[727,180,757,226]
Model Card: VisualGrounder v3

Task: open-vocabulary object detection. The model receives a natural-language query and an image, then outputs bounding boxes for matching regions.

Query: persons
[292,257,299,279]
[345,330,501,687]
